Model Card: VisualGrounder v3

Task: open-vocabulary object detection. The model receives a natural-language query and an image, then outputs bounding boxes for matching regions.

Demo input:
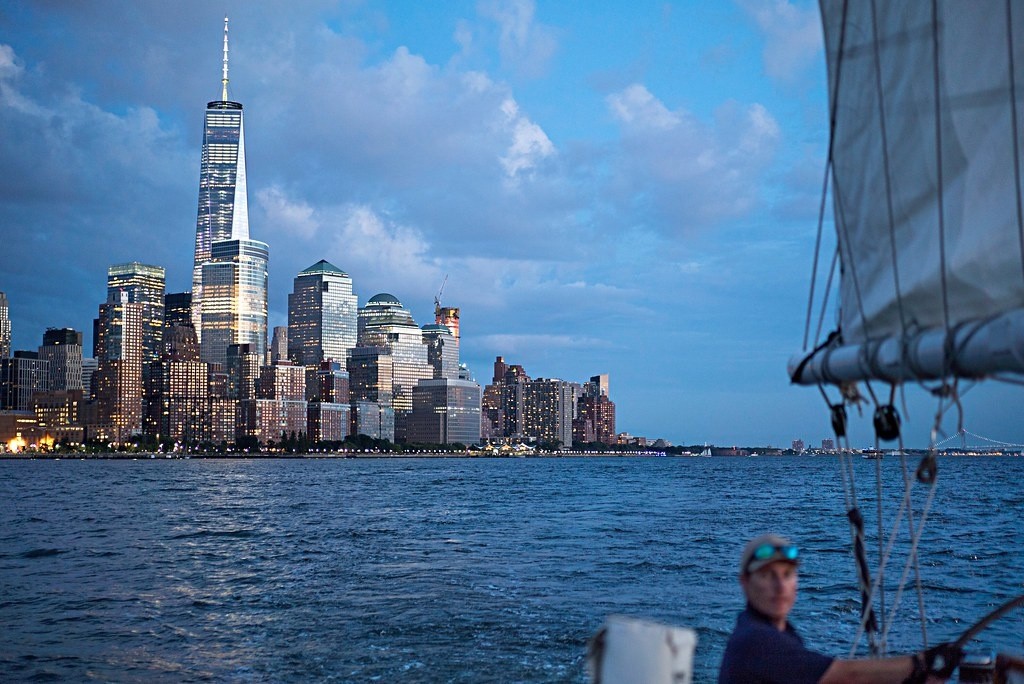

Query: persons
[718,534,967,684]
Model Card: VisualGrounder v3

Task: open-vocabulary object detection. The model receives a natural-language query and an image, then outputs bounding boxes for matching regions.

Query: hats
[743,535,789,580]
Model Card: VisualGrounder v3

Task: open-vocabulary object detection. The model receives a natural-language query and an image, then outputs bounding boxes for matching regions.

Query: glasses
[739,542,799,580]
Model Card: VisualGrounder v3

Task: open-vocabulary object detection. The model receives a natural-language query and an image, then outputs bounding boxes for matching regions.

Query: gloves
[925,642,963,680]
